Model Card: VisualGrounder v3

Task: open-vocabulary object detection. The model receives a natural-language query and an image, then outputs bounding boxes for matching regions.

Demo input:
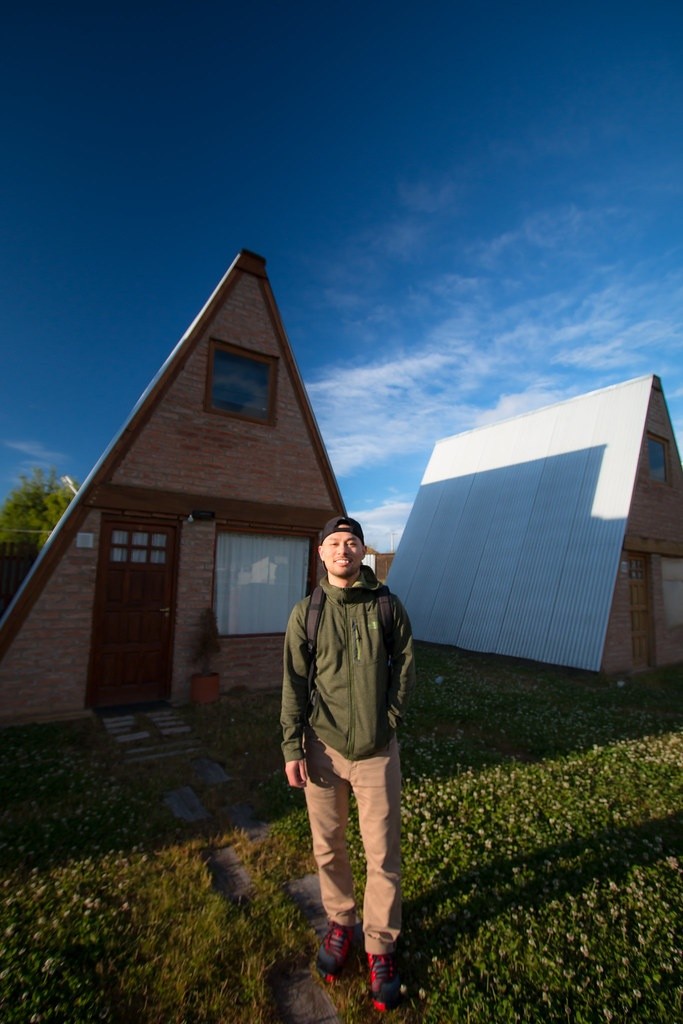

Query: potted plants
[189,606,221,705]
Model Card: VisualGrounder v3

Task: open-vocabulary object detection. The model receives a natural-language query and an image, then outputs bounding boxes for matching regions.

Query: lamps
[192,510,216,519]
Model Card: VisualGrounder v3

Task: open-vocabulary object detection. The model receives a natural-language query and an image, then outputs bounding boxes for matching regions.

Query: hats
[319,516,365,573]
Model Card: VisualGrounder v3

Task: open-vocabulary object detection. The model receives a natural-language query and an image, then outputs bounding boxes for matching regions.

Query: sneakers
[315,920,358,976]
[367,952,401,1013]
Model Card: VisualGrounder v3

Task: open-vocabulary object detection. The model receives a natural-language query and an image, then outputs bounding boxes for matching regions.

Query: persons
[279,517,415,1011]
